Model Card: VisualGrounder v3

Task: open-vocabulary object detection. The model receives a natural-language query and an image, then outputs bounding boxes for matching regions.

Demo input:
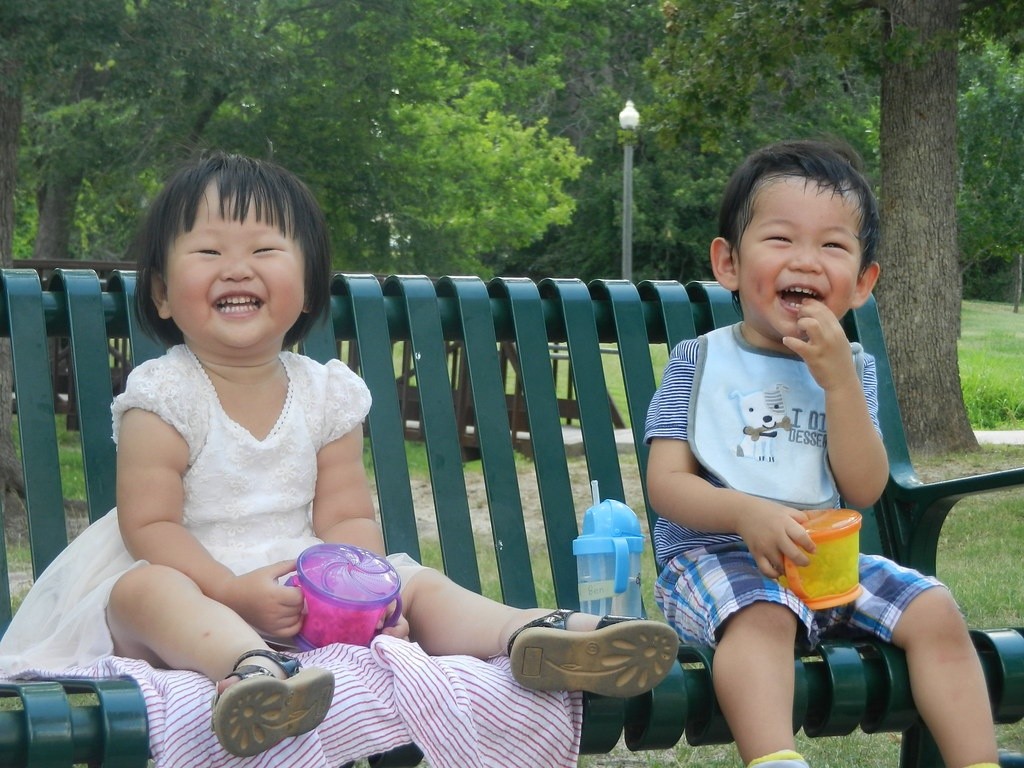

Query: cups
[282,544,401,653]
[780,508,863,611]
[572,481,647,619]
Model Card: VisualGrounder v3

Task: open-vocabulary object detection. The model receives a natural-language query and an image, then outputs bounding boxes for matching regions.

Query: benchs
[1,268,1024,768]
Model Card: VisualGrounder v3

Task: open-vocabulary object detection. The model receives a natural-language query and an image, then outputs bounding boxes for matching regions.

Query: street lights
[619,98,641,283]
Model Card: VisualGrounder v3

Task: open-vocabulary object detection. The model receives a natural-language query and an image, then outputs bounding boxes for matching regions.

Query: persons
[644,139,1000,768]
[0,148,678,758]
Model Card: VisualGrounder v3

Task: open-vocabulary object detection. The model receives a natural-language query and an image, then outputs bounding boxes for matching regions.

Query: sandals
[210,650,335,758]
[509,608,680,698]
